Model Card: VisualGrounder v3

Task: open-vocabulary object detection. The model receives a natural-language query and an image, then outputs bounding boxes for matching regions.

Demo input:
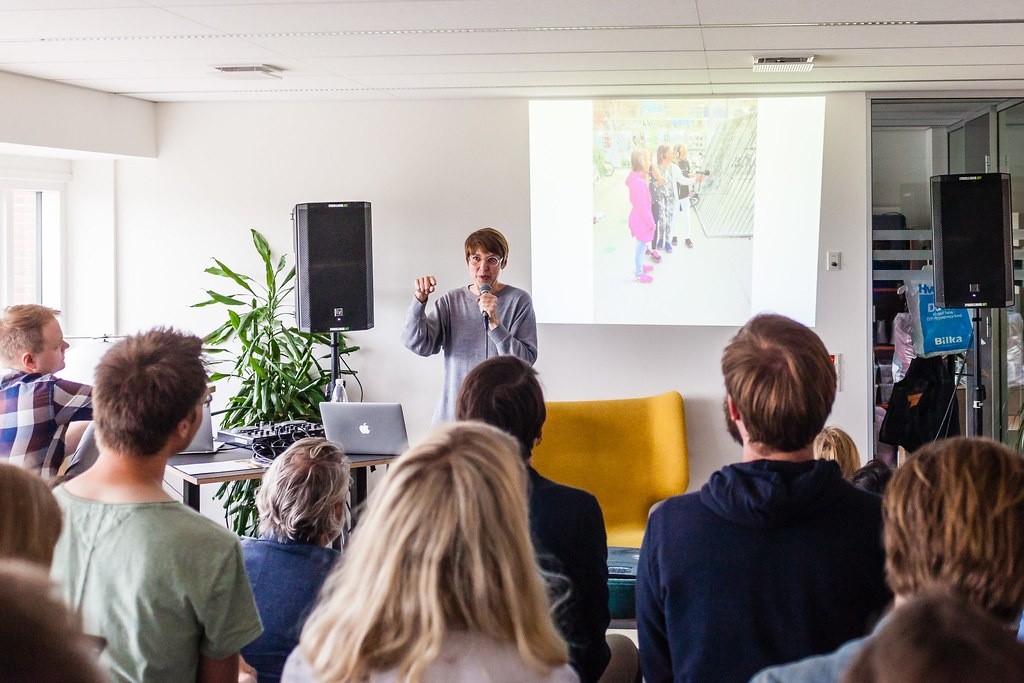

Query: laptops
[176,403,225,454]
[319,402,411,456]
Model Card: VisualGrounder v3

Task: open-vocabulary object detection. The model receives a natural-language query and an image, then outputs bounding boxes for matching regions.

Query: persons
[627,143,705,281]
[403,227,536,422]
[0,308,1024,683]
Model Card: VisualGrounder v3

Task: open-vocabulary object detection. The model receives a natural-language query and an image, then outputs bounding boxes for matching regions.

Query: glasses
[468,255,503,268]
[191,394,212,409]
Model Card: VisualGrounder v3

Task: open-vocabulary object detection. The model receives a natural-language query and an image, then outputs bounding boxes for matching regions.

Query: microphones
[480,284,493,330]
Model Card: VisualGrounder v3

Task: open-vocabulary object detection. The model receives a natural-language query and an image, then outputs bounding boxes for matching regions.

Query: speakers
[290,201,374,333]
[930,173,1016,310]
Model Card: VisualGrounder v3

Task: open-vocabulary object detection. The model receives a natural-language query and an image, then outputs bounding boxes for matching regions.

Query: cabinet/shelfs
[873,288,900,408]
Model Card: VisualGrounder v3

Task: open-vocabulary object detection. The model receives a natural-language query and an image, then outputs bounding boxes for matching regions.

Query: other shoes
[631,235,693,284]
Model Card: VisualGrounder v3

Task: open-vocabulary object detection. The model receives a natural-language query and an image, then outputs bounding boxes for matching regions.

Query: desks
[165,443,402,548]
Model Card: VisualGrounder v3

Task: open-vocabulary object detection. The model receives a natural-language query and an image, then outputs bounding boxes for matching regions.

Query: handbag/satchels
[904,260,974,357]
